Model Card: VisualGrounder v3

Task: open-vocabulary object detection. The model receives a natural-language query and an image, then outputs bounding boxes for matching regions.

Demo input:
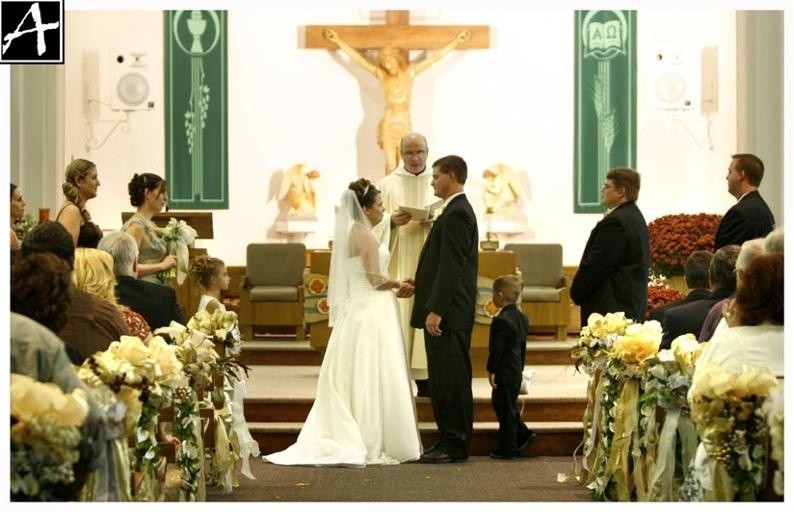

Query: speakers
[113,53,158,111]
[650,42,717,113]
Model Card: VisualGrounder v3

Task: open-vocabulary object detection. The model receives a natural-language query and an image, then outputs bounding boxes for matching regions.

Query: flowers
[644,212,726,282]
[643,288,688,316]
[570,312,776,501]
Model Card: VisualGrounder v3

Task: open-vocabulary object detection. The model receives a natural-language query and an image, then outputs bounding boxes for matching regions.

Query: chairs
[238,242,307,342]
[503,242,570,341]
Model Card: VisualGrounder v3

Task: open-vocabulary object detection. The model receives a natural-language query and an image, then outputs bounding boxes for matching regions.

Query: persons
[321,27,472,175]
[647,228,784,420]
[53,158,103,248]
[10,221,188,394]
[402,155,478,464]
[713,153,776,252]
[120,172,178,286]
[189,255,231,314]
[10,183,26,250]
[486,274,538,460]
[570,167,650,327]
[262,176,425,467]
[375,132,447,395]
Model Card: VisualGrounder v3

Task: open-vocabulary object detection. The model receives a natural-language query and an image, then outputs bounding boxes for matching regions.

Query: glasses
[402,149,427,158]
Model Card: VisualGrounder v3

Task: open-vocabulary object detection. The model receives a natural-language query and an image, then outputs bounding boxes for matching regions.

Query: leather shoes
[424,442,439,454]
[489,448,516,460]
[516,429,537,452]
[418,448,469,464]
